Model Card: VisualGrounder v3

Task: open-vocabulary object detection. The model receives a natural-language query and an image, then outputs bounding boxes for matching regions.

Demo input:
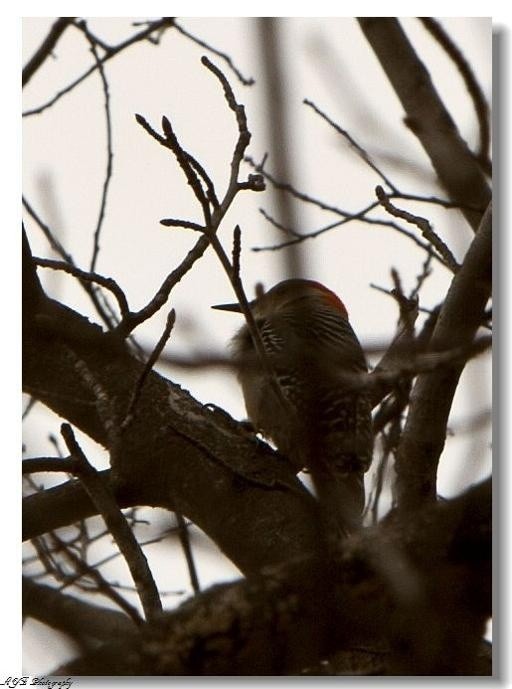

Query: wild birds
[212,277,375,517]
